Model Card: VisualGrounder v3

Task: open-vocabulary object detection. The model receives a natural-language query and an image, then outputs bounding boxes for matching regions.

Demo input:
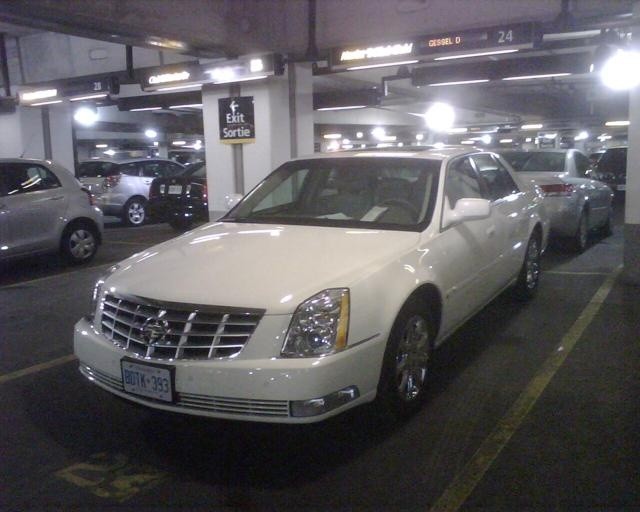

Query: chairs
[364,178,415,224]
